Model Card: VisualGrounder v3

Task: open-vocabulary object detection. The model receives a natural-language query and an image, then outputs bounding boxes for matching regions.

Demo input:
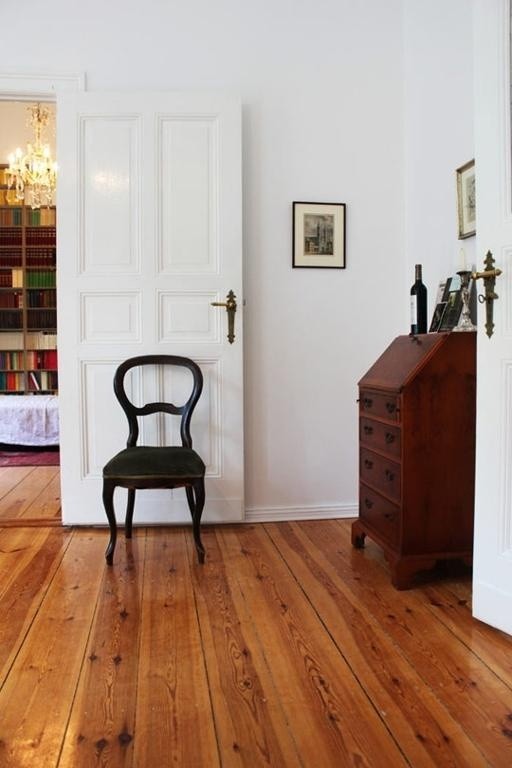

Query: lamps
[4,101,58,211]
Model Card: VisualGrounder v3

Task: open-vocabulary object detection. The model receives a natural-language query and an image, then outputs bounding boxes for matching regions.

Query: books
[1,164,58,395]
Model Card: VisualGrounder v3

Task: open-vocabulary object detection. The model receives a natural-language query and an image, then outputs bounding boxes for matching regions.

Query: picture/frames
[456,158,476,239]
[292,201,346,270]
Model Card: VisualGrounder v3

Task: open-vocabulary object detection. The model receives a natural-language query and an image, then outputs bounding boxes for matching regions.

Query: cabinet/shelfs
[0,163,59,394]
[352,332,477,590]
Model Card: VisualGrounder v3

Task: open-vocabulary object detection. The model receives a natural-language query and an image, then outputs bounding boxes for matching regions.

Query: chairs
[100,355,212,565]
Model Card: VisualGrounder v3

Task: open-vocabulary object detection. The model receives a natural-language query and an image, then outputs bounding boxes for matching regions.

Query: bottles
[410,264,427,334]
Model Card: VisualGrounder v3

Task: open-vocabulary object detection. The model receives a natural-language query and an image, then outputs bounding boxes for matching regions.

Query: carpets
[0,452,59,466]
